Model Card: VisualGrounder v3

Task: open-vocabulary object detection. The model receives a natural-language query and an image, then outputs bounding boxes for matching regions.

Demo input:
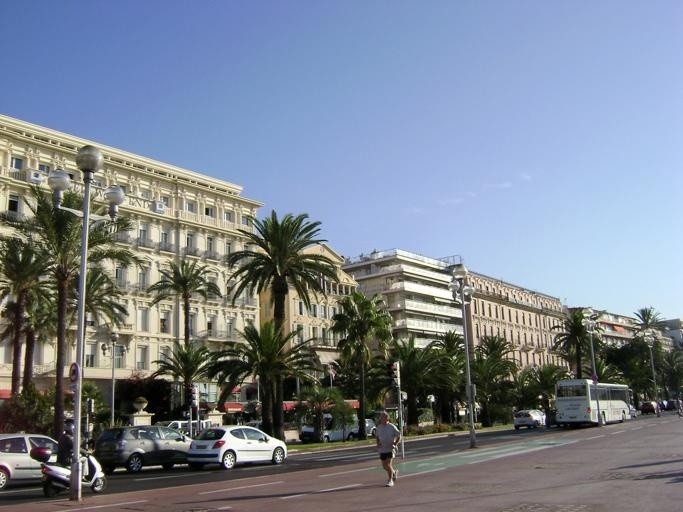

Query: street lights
[643,328,658,400]
[45,143,126,505]
[578,306,602,427]
[446,262,481,450]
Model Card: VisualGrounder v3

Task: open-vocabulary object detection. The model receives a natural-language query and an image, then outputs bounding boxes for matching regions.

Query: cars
[628,398,682,418]
[66,415,96,436]
[186,424,290,471]
[363,419,377,437]
[543,411,566,430]
[512,409,545,431]
[0,430,82,490]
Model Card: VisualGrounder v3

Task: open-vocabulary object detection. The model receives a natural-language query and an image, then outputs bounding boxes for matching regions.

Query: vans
[93,424,195,474]
[152,418,209,438]
[295,412,358,444]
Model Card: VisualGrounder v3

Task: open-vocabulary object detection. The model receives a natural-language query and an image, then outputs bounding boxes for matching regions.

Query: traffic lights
[390,361,400,389]
[189,385,199,408]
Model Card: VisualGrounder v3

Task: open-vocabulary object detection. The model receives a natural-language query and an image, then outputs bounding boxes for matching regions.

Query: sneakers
[386,470,397,487]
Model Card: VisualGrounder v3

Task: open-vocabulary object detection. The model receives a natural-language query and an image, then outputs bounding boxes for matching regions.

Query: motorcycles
[26,443,110,499]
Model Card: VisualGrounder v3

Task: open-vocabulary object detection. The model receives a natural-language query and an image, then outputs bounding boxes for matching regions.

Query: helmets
[64,422,74,434]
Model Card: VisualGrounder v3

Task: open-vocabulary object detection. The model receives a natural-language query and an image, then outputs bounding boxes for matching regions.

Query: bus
[550,379,634,428]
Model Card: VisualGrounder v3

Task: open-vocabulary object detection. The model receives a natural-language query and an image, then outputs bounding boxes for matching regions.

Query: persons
[375,412,401,487]
[57,422,92,482]
